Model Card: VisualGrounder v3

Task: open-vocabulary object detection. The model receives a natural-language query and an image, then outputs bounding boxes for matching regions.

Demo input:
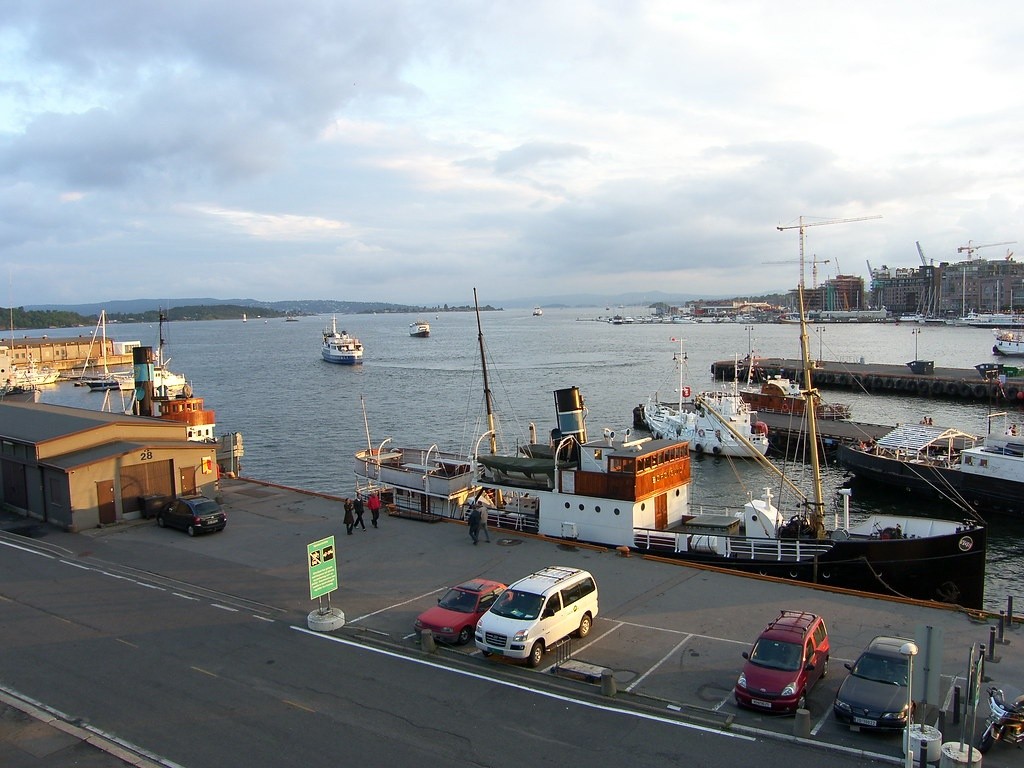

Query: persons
[353,493,367,531]
[919,416,933,425]
[468,504,482,545]
[478,501,491,543]
[343,498,354,535]
[367,491,380,528]
[867,438,875,447]
[1006,426,1012,435]
[1012,424,1016,436]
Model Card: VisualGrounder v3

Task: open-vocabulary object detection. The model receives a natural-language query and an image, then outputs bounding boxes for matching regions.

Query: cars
[155,494,228,537]
[414,578,514,646]
[833,634,914,733]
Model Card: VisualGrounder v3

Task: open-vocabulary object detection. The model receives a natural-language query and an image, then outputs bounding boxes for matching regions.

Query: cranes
[777,214,884,310]
[957,240,1018,262]
[916,241,928,266]
[865,259,874,278]
[834,257,841,275]
[761,254,830,289]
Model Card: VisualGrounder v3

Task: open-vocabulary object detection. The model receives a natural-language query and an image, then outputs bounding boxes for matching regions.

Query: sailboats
[9,301,62,386]
[80,305,194,400]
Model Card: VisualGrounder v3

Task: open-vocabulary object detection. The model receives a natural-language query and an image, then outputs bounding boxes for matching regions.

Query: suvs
[735,609,830,716]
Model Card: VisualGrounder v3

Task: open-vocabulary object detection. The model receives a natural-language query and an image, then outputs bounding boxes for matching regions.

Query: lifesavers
[936,492,946,503]
[648,426,652,433]
[782,403,788,411]
[711,365,1019,401]
[756,421,768,436]
[390,448,401,461]
[972,496,984,508]
[695,444,703,452]
[643,419,648,426]
[84,382,87,386]
[714,458,721,464]
[656,432,662,439]
[682,386,690,397]
[713,446,721,453]
[698,429,705,437]
[183,385,191,397]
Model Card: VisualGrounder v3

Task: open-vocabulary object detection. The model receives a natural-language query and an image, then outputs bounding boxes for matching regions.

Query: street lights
[744,325,754,354]
[911,328,920,362]
[815,326,826,362]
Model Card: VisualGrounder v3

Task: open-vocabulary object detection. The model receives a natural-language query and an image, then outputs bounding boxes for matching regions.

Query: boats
[642,336,769,459]
[607,312,756,324]
[321,313,363,365]
[992,327,1024,358]
[532,307,543,316]
[99,384,245,477]
[898,265,1024,330]
[836,423,1024,525]
[351,281,986,610]
[409,320,431,338]
[739,349,852,419]
[778,311,815,324]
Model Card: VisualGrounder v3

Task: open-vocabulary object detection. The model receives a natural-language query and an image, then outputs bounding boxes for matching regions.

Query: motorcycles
[977,686,1024,756]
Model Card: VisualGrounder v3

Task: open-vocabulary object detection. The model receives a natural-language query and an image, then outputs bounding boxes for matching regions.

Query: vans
[474,566,598,668]
